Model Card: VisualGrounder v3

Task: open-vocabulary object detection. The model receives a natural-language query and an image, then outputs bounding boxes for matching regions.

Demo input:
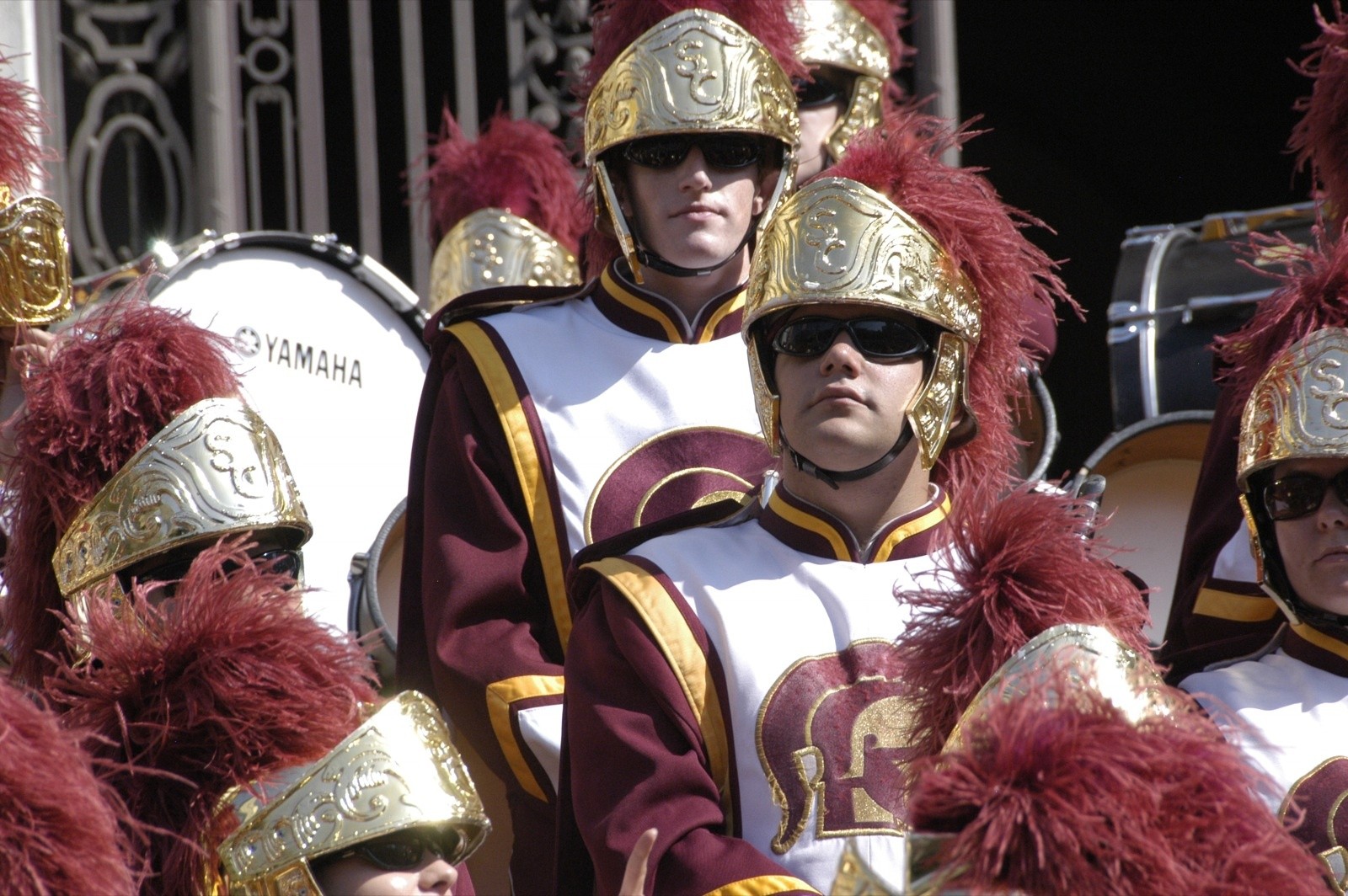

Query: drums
[1107,202,1323,429]
[1070,411,1216,666]
[67,229,430,645]
[349,495,409,692]
[1002,367,1058,499]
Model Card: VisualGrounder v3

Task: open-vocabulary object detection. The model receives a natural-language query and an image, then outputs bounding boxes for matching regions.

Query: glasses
[766,312,935,360]
[134,553,306,606]
[790,73,847,112]
[616,133,765,173]
[1252,464,1348,522]
[313,824,468,872]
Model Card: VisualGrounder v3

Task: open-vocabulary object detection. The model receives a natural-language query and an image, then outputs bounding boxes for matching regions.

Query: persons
[83,720,492,896]
[412,44,799,896]
[19,422,311,632]
[1178,366,1348,870]
[561,224,1025,896]
[758,0,903,190]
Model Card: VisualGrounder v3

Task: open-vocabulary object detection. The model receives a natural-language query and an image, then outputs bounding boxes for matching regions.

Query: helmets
[0,302,314,664]
[45,542,493,896]
[424,112,589,312]
[1232,9,1348,628]
[0,45,73,325]
[781,0,909,164]
[743,112,1088,469]
[581,0,802,287]
[915,484,1222,761]
